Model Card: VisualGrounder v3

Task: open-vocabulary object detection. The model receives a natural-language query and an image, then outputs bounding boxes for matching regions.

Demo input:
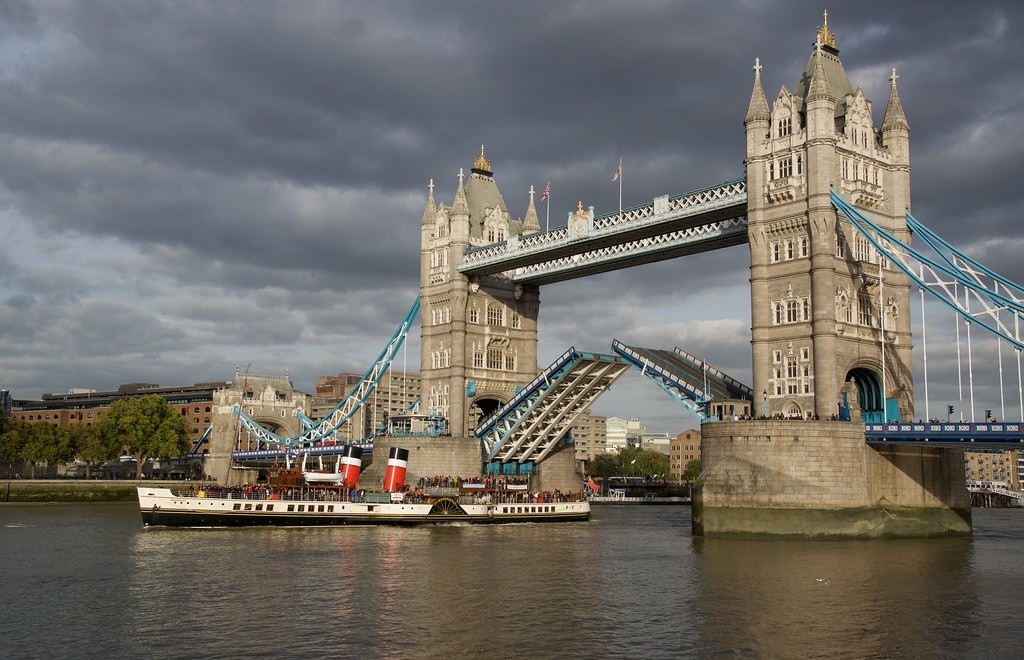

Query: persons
[888,416,997,423]
[171,476,587,505]
[733,410,839,421]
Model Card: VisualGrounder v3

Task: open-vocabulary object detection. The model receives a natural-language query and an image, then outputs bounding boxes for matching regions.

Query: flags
[611,166,622,183]
[587,478,600,491]
[540,184,550,202]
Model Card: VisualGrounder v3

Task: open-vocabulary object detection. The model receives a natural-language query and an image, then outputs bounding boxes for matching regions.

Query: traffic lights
[950,405,954,415]
[988,410,991,419]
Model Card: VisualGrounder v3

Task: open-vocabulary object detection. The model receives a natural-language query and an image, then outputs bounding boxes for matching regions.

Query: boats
[138,484,592,524]
